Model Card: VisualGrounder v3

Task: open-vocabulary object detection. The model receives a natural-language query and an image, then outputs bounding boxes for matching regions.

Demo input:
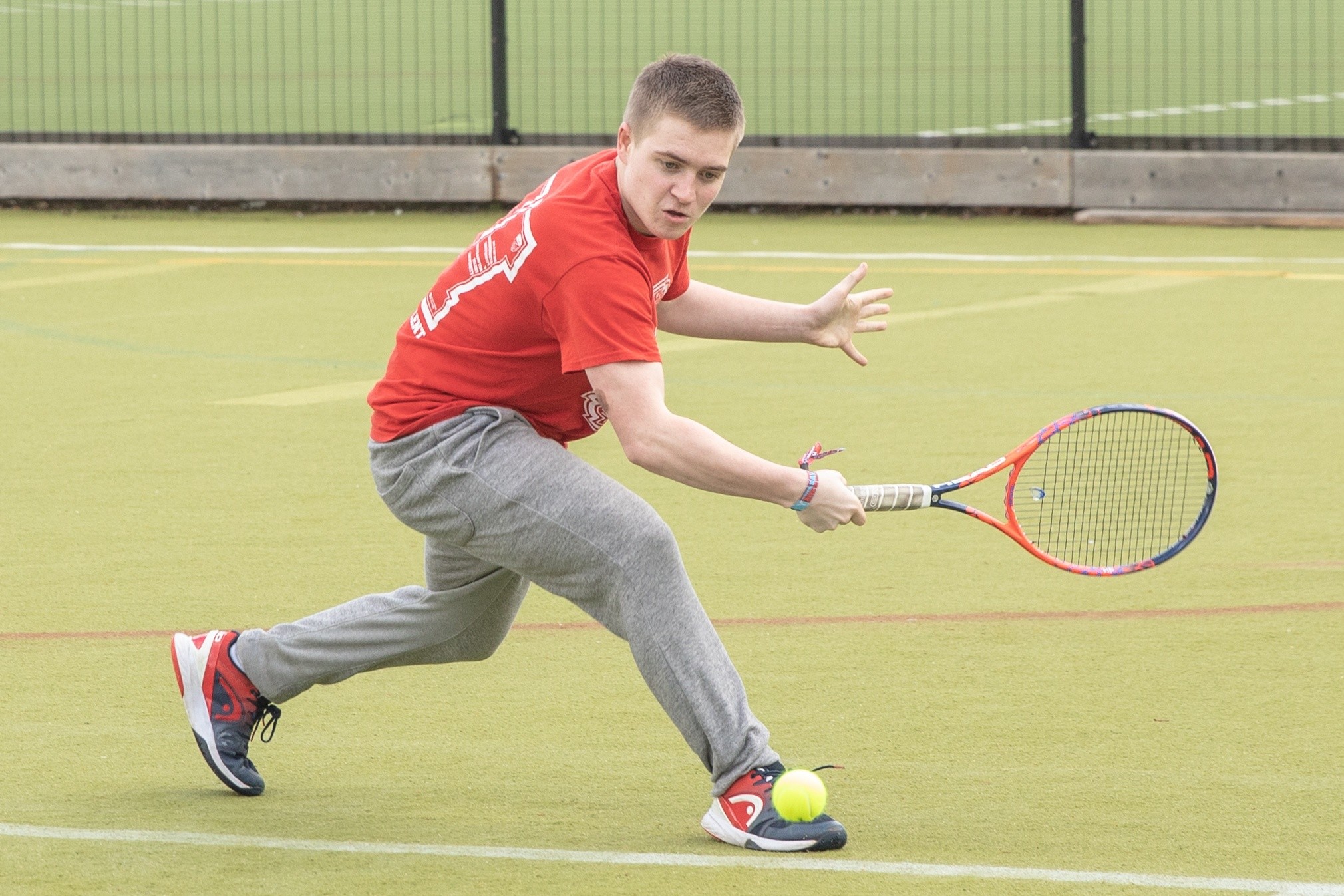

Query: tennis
[772,767,825,820]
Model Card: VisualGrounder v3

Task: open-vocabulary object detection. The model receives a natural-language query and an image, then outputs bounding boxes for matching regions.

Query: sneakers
[699,761,849,854]
[169,628,282,797]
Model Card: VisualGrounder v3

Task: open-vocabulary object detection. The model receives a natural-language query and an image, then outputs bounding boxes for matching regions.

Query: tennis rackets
[847,402,1215,578]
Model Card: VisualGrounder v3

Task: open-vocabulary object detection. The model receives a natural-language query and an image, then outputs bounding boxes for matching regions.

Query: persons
[169,55,895,855]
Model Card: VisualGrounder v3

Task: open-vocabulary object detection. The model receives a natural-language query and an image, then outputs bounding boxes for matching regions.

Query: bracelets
[791,446,846,515]
